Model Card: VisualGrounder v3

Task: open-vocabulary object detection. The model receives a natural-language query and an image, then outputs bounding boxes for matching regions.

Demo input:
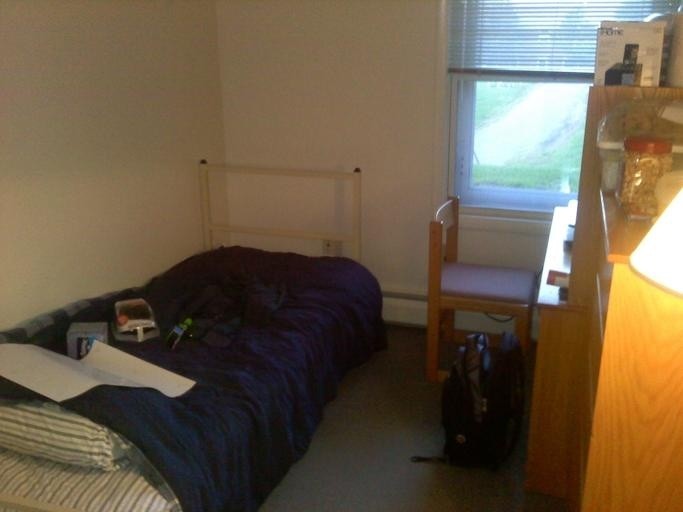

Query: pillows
[0,391,138,473]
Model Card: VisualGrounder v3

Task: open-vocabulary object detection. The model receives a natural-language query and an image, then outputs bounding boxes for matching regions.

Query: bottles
[614,135,673,220]
[165,316,193,351]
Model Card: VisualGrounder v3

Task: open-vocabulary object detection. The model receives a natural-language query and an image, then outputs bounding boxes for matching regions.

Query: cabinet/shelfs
[529,86,683,512]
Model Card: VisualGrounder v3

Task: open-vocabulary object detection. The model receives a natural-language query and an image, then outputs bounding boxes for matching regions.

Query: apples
[117,315,128,325]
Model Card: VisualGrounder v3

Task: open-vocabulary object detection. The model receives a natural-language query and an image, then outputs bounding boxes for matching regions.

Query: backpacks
[441,330,533,473]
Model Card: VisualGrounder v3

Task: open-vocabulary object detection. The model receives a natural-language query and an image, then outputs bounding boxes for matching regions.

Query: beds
[0,158,381,512]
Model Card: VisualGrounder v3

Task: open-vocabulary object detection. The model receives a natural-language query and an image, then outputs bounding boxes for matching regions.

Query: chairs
[426,195,535,376]
